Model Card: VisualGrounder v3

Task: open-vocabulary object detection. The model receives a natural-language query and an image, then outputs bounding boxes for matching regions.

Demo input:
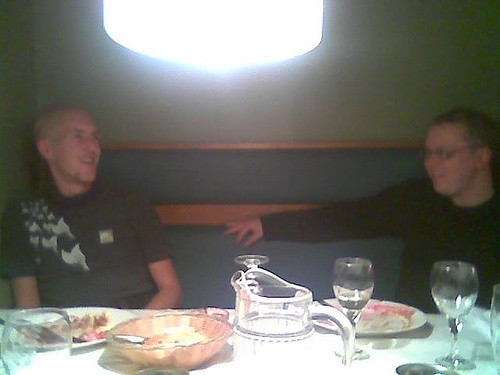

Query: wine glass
[234,254,270,268]
[429,260,480,371]
[333,256,375,360]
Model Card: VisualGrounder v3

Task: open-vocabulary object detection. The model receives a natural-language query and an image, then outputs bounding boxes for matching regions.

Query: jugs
[231,267,356,375]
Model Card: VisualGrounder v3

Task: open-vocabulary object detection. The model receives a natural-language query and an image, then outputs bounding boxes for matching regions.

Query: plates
[310,298,427,338]
[26,306,136,351]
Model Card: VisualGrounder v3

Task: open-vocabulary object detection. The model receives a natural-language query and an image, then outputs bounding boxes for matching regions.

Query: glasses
[419,142,480,163]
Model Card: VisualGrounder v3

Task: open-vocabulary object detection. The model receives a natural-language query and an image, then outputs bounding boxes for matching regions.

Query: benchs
[34,139,434,305]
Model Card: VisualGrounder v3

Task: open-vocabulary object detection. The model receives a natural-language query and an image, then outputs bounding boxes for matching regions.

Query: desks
[0,308,500,375]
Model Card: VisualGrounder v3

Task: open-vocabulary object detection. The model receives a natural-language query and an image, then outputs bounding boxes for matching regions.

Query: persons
[0,103,182,310]
[226,107,500,316]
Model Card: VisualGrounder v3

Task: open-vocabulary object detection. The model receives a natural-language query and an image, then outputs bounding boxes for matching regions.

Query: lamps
[101,0,324,66]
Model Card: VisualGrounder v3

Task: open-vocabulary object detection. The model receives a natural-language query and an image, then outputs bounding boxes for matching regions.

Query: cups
[0,307,73,375]
[488,283,500,375]
